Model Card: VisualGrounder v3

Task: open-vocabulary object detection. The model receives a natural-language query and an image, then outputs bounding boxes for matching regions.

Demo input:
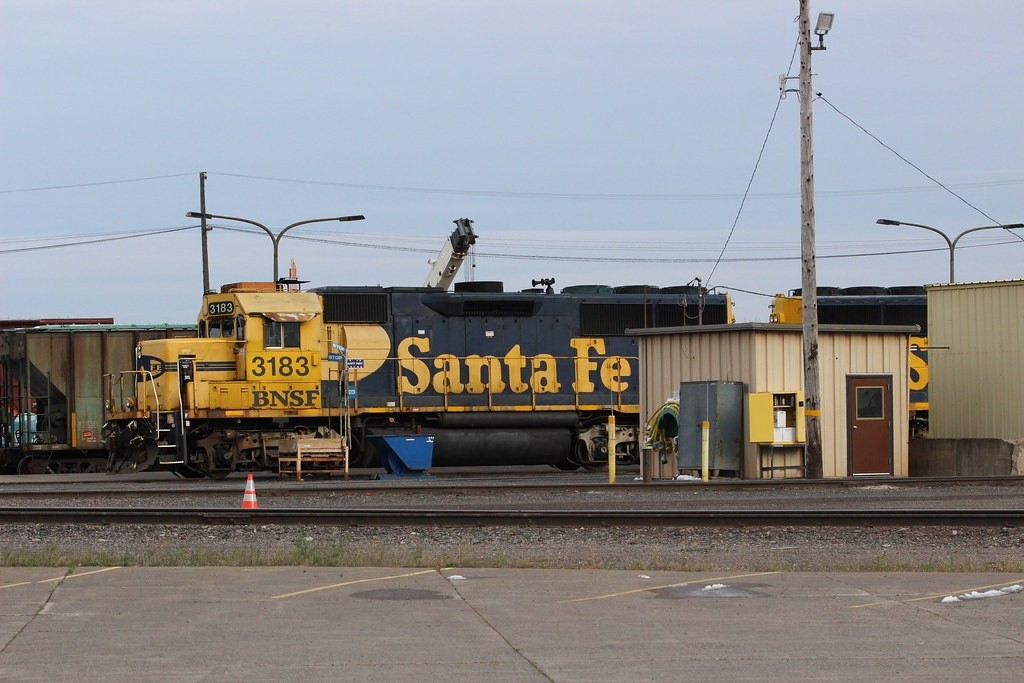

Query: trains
[0,323,242,474]
[101,277,928,488]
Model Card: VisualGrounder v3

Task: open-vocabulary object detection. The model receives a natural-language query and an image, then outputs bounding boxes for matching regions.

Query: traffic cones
[242,472,258,510]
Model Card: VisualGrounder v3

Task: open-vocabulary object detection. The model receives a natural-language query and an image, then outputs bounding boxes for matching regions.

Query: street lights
[877,219,1024,282]
[183,211,366,290]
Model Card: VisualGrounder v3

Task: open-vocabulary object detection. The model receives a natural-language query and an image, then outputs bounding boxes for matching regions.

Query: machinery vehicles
[423,218,479,292]
